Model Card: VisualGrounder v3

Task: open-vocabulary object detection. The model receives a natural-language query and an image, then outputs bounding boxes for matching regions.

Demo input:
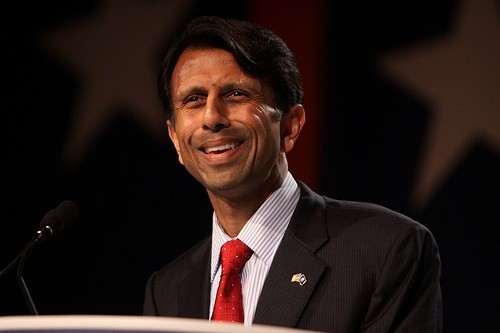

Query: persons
[143,15,443,333]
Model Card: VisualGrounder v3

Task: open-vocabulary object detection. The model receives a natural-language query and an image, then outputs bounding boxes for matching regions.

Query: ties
[211,239,253,324]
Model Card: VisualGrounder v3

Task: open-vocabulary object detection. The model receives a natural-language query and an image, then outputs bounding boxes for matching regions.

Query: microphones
[18,200,79,315]
[0,209,55,276]
